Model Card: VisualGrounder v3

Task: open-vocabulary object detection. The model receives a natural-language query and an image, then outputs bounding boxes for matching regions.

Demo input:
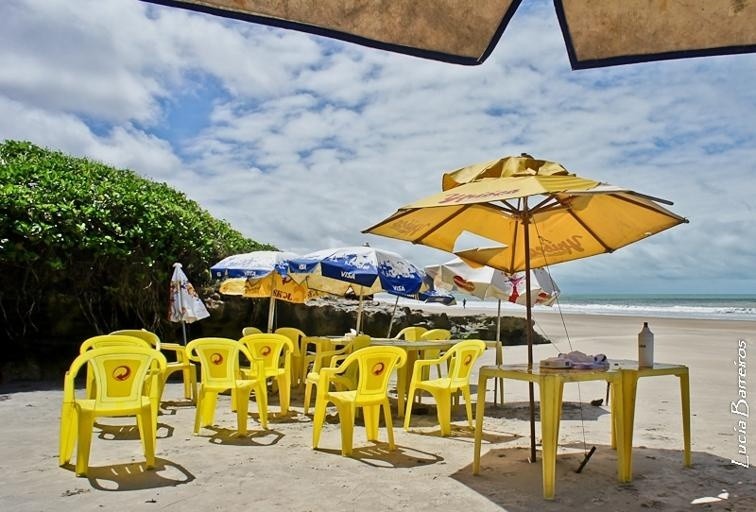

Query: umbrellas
[168,262,210,346]
[137,0,756,71]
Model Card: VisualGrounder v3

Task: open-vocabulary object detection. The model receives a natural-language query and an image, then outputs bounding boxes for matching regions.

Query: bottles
[638,321,654,368]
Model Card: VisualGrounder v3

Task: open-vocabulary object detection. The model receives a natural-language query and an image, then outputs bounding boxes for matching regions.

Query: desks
[472,359,692,502]
[370,338,505,418]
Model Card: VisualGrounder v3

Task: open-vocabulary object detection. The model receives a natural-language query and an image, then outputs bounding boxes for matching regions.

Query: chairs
[56,328,486,476]
